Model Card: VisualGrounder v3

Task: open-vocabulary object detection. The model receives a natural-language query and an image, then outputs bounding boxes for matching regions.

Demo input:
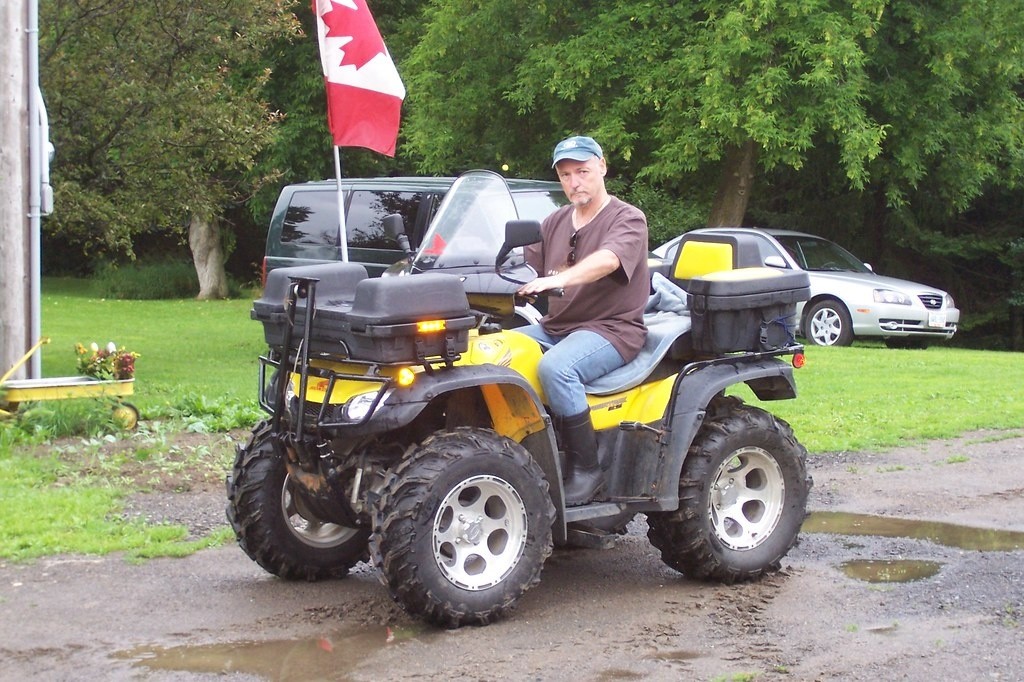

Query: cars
[651,229,960,347]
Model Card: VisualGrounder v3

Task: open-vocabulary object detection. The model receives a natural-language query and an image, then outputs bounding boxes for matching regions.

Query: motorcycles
[224,168,816,623]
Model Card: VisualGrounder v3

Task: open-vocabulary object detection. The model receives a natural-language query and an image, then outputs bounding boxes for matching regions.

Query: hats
[551,136,604,169]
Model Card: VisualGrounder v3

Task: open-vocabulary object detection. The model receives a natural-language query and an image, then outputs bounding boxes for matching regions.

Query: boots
[555,405,608,507]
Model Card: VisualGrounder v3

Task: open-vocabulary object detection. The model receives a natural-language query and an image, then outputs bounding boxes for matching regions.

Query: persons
[515,136,649,507]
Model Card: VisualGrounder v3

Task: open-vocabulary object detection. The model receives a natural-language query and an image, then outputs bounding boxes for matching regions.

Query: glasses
[566,232,577,266]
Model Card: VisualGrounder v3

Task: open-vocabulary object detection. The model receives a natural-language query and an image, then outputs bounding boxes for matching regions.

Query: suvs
[263,177,661,316]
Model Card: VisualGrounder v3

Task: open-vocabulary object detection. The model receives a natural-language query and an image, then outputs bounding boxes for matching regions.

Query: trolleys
[0,337,139,431]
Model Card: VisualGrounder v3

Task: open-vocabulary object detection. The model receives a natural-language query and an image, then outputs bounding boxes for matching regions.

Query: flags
[313,0,406,157]
